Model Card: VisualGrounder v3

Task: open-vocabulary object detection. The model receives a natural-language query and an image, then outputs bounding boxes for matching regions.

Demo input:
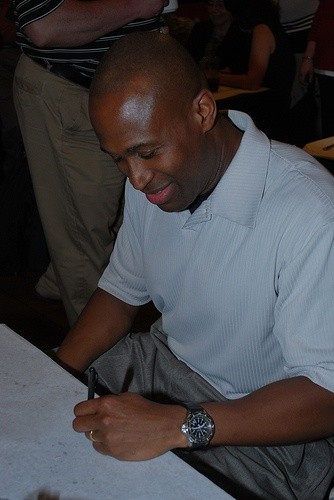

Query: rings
[90,431,94,441]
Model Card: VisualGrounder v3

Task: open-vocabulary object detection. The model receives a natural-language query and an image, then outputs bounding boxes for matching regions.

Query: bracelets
[302,56,314,59]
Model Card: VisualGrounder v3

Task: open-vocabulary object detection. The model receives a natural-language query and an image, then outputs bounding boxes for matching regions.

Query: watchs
[179,402,214,448]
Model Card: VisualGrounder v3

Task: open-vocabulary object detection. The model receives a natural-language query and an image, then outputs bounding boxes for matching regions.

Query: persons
[185,0,297,141]
[273,0,322,140]
[53,33,334,500]
[300,0,334,138]
[13,0,169,336]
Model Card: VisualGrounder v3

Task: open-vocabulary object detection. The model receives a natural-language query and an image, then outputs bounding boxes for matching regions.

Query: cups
[162,0,178,14]
[201,56,219,92]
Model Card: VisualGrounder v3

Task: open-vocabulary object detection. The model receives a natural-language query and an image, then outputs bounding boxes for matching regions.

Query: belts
[28,55,95,91]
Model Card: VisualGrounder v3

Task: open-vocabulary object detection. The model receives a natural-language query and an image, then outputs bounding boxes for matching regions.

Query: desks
[210,83,277,120]
[0,321,236,500]
